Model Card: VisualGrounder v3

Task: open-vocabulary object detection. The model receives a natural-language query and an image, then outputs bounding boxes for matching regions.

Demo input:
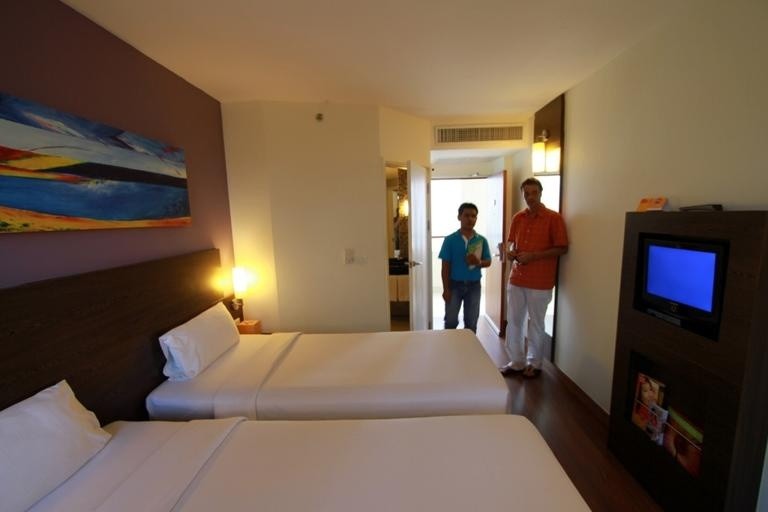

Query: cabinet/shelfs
[390,275,410,302]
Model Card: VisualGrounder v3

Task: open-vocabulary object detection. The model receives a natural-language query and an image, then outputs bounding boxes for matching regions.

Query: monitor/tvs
[639,239,726,324]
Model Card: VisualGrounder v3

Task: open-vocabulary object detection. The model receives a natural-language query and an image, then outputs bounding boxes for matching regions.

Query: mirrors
[392,191,400,258]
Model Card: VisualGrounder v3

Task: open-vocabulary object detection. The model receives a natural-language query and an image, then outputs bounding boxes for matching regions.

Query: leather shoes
[499,365,523,376]
[522,365,542,378]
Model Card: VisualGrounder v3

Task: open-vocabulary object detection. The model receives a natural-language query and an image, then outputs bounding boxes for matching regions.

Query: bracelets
[477,260,482,266]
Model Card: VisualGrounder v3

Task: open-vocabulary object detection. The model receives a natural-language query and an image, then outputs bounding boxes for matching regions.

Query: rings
[518,256,520,260]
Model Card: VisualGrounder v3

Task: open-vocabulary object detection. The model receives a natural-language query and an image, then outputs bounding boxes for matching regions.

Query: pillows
[159,302,240,381]
[0,379,113,512]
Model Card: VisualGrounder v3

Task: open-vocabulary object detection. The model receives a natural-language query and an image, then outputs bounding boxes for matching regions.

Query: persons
[438,202,492,335]
[498,175,570,381]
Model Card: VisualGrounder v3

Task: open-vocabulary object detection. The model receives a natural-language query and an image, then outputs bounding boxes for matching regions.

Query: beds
[145,328,508,421]
[28,413,594,512]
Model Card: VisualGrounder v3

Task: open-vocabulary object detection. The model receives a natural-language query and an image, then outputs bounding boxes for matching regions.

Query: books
[633,370,707,476]
[466,239,485,271]
